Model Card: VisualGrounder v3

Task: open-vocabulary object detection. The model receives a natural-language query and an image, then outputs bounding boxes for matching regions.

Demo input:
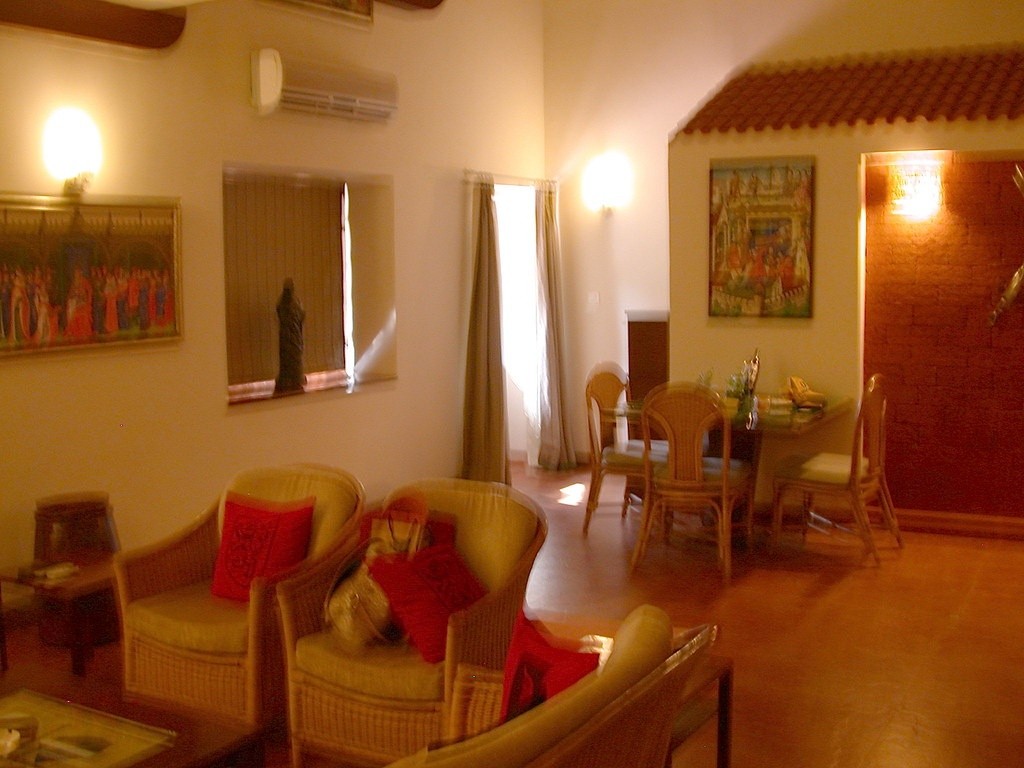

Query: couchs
[112,463,365,738]
[276,477,549,768]
[385,603,719,768]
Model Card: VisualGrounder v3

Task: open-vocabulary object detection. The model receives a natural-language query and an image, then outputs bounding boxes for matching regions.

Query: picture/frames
[257,0,374,32]
[0,194,185,358]
[709,156,815,319]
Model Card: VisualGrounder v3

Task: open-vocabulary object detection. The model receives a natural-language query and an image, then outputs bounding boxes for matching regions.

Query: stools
[33,500,120,642]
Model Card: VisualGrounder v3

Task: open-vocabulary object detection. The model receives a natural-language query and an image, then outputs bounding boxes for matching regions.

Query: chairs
[769,373,905,568]
[581,371,670,535]
[629,381,752,587]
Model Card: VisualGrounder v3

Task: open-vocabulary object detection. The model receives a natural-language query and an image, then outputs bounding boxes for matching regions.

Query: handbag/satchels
[324,538,410,653]
[361,509,455,555]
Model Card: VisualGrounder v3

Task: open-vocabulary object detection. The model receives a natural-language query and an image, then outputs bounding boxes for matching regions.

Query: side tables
[664,655,734,767]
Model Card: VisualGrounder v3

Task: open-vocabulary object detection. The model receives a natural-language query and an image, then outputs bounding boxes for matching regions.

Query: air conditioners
[250,47,400,125]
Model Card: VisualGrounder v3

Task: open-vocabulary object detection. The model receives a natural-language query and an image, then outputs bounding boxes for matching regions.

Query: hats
[381,486,428,530]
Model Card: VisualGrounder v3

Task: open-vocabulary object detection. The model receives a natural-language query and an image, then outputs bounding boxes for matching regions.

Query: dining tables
[623,390,852,549]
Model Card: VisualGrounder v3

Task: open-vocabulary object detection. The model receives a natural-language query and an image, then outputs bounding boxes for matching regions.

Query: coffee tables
[0,545,117,675]
[0,665,262,768]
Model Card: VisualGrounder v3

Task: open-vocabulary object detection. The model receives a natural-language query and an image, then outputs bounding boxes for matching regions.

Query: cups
[764,392,793,416]
